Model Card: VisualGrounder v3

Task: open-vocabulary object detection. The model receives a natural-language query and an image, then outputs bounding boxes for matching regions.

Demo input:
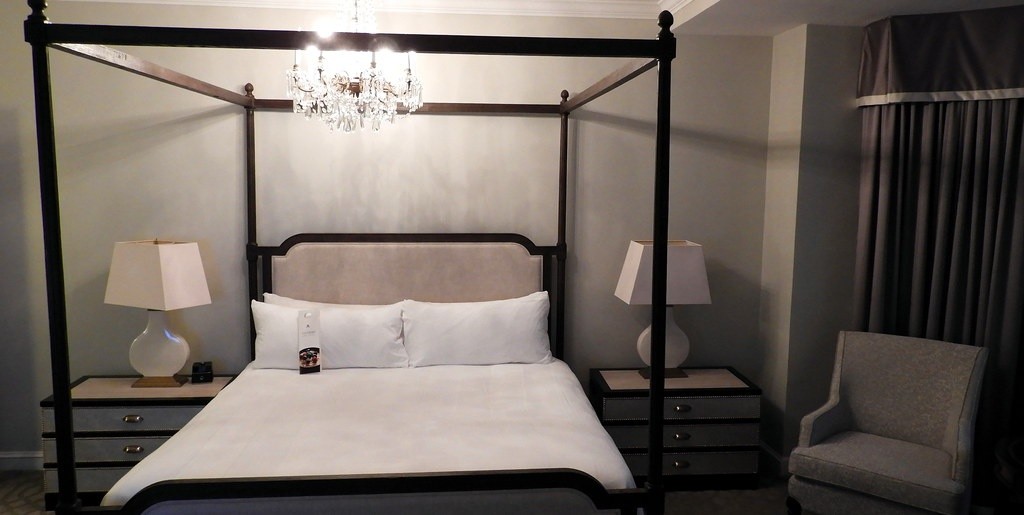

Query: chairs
[784,329,990,515]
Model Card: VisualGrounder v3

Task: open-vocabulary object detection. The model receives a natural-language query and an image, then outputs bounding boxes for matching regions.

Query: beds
[21,0,677,515]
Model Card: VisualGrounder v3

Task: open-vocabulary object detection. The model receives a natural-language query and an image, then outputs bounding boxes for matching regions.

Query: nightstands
[38,372,239,513]
[589,365,764,491]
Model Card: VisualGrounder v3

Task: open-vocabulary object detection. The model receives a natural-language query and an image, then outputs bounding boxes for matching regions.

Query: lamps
[286,0,425,137]
[103,237,214,390]
[613,239,713,381]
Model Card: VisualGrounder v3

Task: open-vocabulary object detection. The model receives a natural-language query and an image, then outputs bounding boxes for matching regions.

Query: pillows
[250,299,409,369]
[403,290,556,369]
[263,292,389,312]
